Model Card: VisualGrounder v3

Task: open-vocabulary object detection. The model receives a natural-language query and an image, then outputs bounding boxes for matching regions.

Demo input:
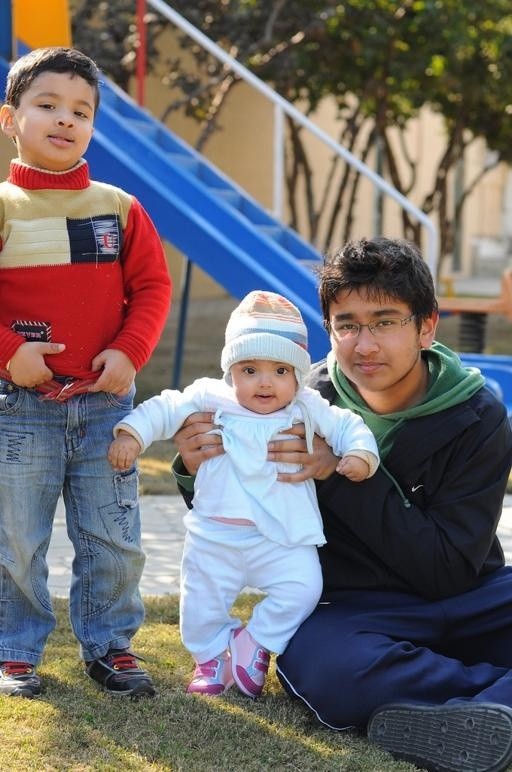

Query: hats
[220,290,311,374]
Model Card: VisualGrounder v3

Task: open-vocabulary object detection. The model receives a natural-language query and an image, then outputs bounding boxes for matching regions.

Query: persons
[174,235,512,770]
[108,290,380,700]
[0,45,172,699]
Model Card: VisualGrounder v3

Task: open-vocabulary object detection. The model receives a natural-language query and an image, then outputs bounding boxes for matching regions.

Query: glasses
[330,313,417,339]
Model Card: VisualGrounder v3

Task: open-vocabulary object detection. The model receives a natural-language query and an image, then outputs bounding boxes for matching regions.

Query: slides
[0,38,512,421]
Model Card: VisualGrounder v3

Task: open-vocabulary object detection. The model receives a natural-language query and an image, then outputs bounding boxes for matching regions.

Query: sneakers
[84,646,155,699]
[228,628,271,698]
[187,651,232,696]
[0,661,42,698]
[365,698,512,771]
